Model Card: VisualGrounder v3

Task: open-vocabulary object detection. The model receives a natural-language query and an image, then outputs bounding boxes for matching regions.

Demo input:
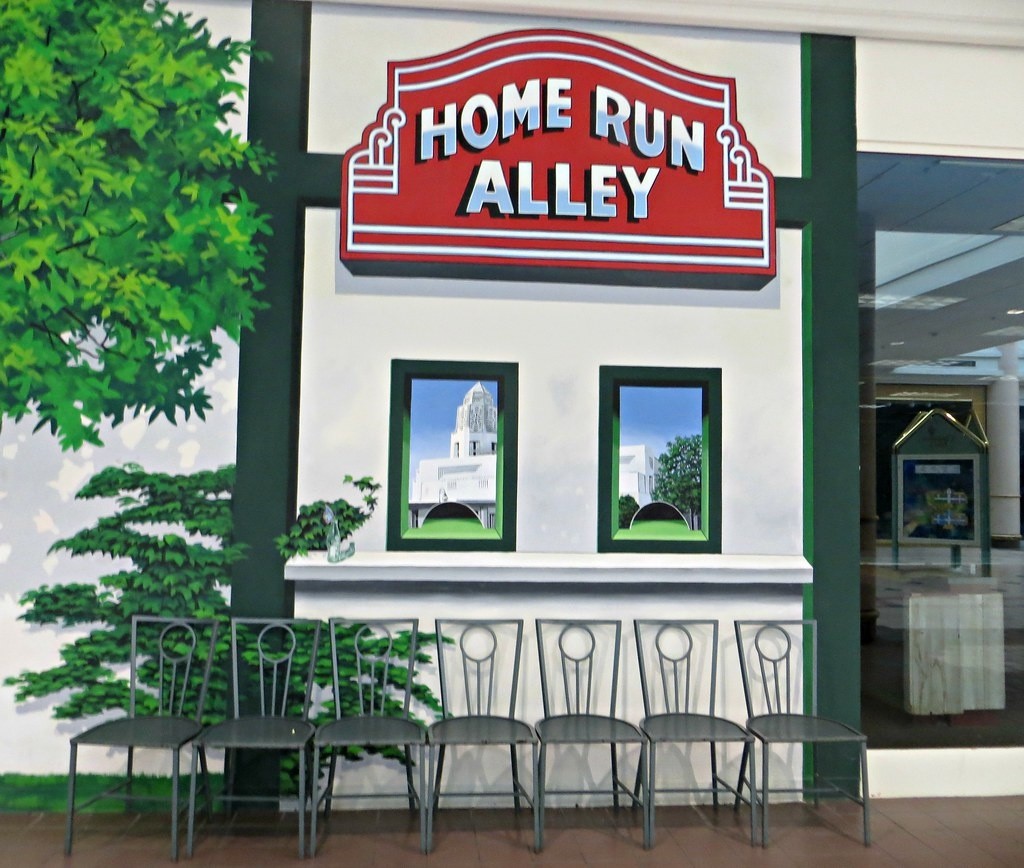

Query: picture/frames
[593,365,722,555]
[383,354,526,551]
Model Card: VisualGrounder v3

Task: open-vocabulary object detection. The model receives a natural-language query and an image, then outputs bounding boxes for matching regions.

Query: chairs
[526,616,645,848]
[632,619,754,849]
[420,613,535,854]
[184,614,325,860]
[727,616,874,848]
[308,614,423,857]
[64,614,220,862]
